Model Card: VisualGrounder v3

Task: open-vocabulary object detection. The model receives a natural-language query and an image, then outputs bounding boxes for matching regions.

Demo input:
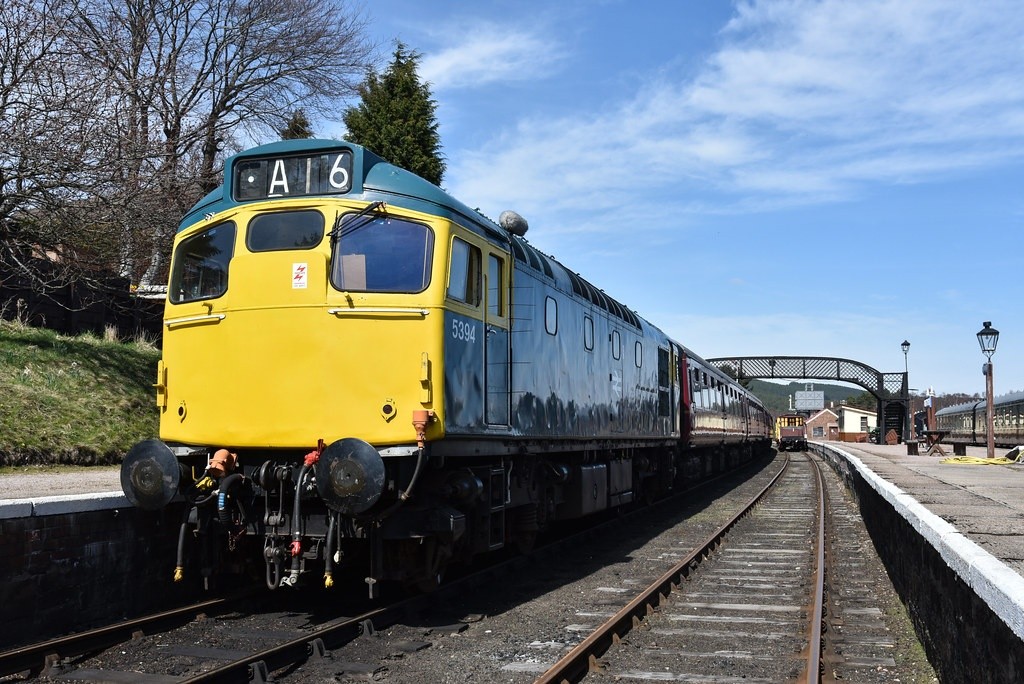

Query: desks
[922,430,951,456]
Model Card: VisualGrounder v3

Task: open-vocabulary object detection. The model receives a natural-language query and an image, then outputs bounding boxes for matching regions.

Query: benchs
[953,441,969,456]
[907,440,919,456]
[918,439,935,448]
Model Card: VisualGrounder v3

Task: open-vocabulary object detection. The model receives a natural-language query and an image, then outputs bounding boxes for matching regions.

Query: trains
[121,136,774,593]
[775,413,808,452]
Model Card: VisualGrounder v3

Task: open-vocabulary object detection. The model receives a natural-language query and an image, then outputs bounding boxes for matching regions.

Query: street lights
[975,321,1000,460]
[900,339,911,444]
